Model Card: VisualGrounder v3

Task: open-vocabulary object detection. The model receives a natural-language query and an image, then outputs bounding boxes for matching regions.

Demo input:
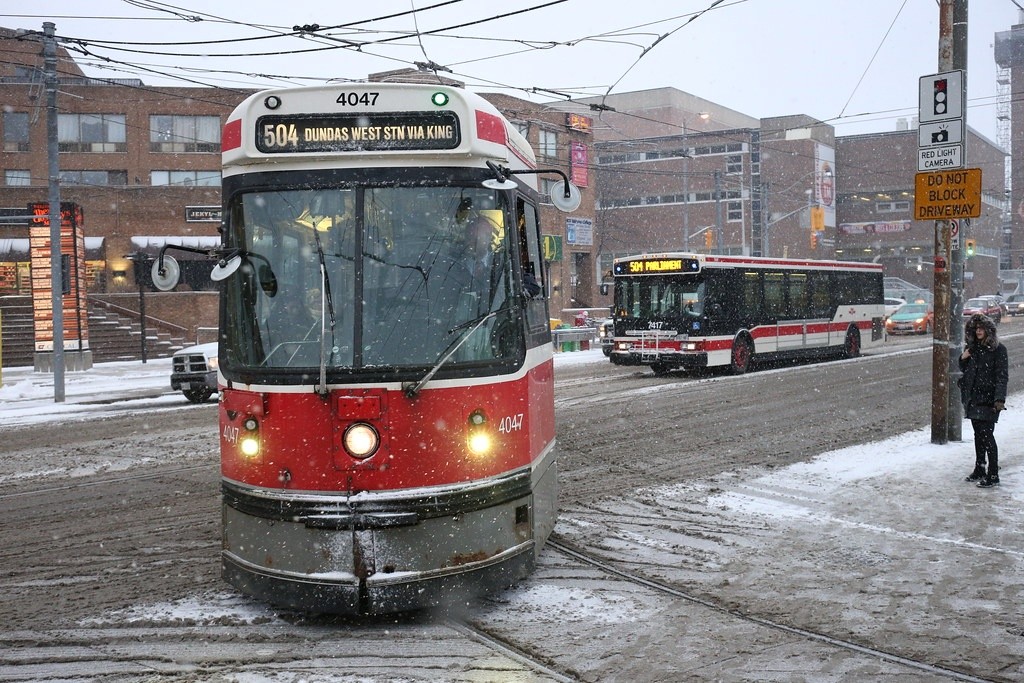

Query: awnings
[130,235,220,260]
[0,237,106,261]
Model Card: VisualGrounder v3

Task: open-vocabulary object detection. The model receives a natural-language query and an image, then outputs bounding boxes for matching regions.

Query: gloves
[991,399,1007,415]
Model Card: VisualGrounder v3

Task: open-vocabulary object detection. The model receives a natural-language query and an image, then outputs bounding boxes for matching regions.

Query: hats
[305,289,323,304]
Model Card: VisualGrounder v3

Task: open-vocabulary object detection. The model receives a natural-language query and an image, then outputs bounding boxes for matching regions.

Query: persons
[957,313,1009,487]
[996,292,1000,295]
[448,218,541,298]
[976,293,981,297]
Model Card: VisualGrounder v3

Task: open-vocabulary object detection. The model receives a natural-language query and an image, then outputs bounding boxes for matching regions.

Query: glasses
[975,326,984,329]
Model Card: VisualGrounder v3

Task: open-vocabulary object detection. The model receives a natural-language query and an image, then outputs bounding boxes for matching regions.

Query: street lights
[124,254,147,364]
[763,170,831,257]
[682,112,710,251]
[959,210,1011,247]
[724,155,746,256]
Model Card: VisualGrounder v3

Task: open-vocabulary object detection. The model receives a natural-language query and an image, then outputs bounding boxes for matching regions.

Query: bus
[600,252,885,375]
[151,80,581,624]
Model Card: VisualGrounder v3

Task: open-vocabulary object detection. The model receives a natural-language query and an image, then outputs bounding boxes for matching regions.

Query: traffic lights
[966,239,976,259]
[934,81,948,114]
[811,233,817,250]
[704,230,713,248]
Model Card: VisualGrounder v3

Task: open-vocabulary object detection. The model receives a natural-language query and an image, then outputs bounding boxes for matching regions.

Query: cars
[963,297,1001,326]
[1005,293,1024,316]
[600,301,705,356]
[885,299,934,335]
[979,295,1009,316]
[884,298,907,319]
[168,339,222,403]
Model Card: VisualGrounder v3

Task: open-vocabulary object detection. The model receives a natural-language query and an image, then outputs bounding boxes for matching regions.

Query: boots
[965,461,987,482]
[975,467,1000,488]
